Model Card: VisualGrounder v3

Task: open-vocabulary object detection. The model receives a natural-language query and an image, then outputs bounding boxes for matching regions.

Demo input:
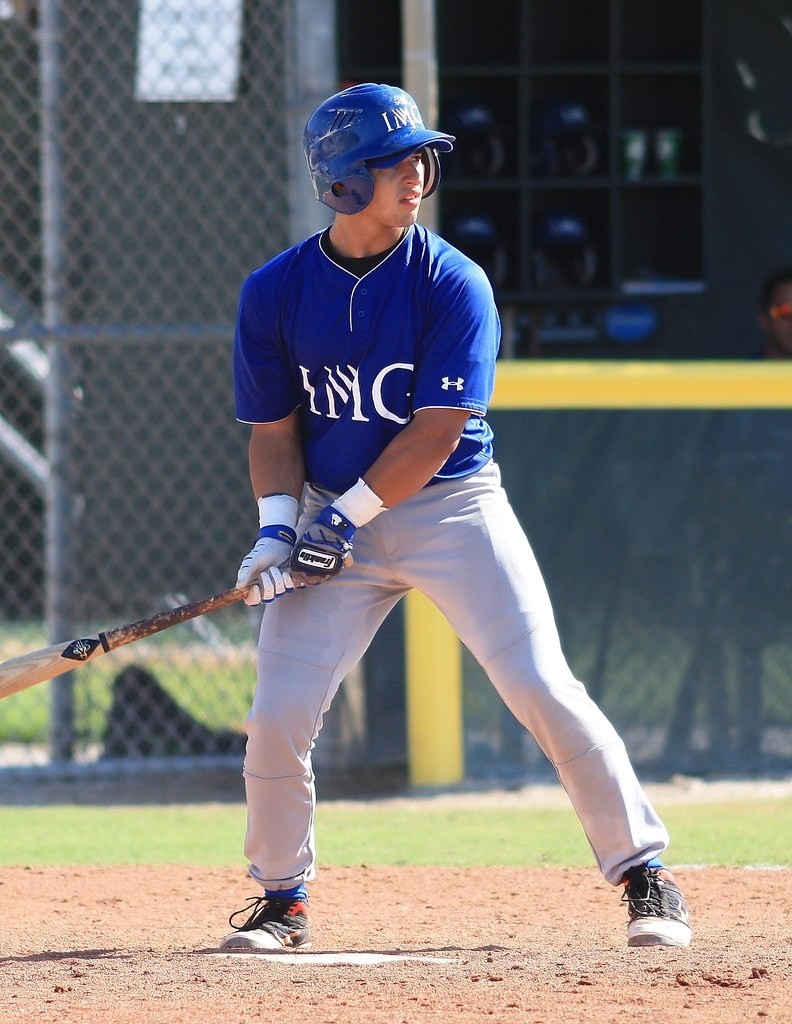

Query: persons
[219,83,691,949]
[695,266,792,648]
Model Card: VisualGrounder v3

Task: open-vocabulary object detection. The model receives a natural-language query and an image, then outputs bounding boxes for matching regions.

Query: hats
[368,138,453,168]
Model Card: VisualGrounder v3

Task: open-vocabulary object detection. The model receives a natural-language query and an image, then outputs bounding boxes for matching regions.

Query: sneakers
[621,859,690,948]
[220,896,312,949]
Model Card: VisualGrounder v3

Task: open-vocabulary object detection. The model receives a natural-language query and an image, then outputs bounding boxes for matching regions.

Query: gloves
[235,524,297,607]
[288,505,356,587]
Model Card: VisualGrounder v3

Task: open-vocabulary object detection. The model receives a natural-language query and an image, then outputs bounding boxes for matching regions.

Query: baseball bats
[2,550,356,703]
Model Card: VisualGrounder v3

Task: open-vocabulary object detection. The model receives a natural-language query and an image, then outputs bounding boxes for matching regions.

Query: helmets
[303,84,456,215]
